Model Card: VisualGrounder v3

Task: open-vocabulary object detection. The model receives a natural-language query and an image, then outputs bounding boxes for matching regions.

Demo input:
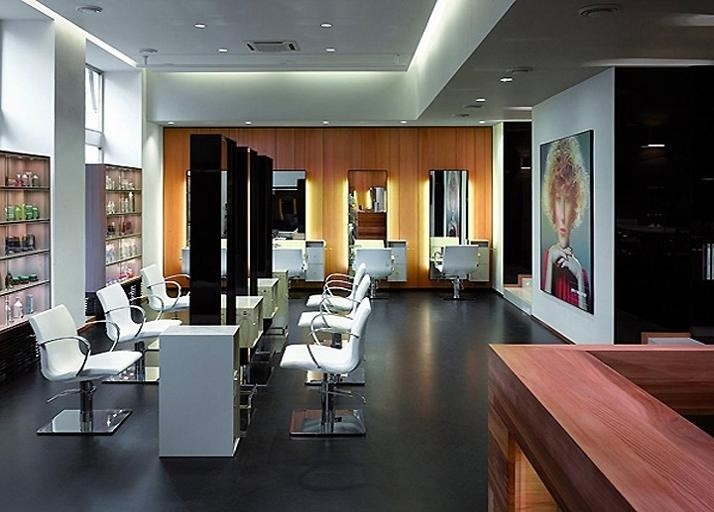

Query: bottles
[14,171,41,187]
[12,297,24,319]
[26,294,34,314]
[6,205,39,220]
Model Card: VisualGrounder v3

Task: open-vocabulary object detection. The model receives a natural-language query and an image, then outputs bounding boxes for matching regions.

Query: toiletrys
[11,274,38,285]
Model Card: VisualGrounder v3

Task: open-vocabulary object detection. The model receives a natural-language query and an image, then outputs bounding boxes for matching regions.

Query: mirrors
[189,134,273,326]
[273,171,305,240]
[429,169,469,280]
[347,169,387,281]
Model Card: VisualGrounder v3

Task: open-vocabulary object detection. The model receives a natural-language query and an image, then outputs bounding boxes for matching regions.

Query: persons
[542,137,588,309]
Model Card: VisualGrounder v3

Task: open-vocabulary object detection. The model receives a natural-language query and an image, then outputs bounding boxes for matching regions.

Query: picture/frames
[540,130,594,315]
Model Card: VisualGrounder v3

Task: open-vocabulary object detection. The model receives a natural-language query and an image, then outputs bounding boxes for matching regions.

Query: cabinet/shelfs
[159,269,289,457]
[306,240,326,282]
[387,239,407,282]
[85,164,143,320]
[0,149,51,389]
[468,240,490,282]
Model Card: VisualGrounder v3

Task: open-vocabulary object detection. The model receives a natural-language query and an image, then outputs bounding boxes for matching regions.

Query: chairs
[179,248,190,296]
[29,248,190,436]
[352,248,394,299]
[430,237,459,262]
[351,239,386,248]
[273,239,305,248]
[279,263,371,439]
[432,245,480,300]
[272,249,304,299]
